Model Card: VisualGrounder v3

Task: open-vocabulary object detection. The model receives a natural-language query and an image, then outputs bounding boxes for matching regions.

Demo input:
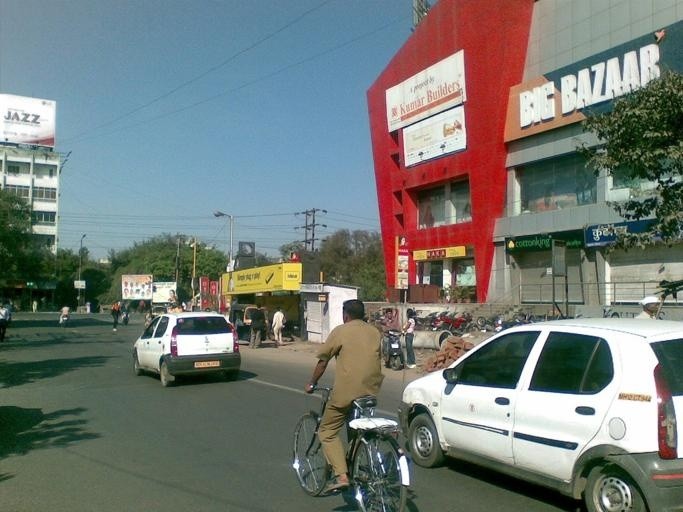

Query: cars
[397,317,682,512]
[132,311,240,386]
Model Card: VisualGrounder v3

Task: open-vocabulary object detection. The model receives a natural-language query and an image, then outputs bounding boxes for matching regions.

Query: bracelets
[307,383,317,390]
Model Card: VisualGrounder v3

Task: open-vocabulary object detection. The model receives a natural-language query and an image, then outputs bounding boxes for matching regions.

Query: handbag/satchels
[283,316,287,324]
[254,320,264,330]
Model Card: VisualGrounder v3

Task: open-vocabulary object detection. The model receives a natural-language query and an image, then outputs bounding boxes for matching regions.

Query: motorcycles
[414,306,539,335]
[380,328,404,370]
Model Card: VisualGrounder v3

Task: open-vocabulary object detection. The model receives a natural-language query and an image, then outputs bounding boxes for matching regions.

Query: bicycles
[291,386,410,510]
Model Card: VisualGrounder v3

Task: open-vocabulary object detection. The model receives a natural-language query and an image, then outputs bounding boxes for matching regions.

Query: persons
[32,301,37,312]
[110,301,121,331]
[124,289,129,297]
[382,307,404,369]
[304,299,386,494]
[633,296,661,320]
[166,289,176,302]
[139,299,145,313]
[247,303,266,348]
[270,307,286,346]
[0,304,9,342]
[402,309,416,368]
[398,236,407,250]
[144,276,151,288]
[59,305,70,324]
[121,303,130,316]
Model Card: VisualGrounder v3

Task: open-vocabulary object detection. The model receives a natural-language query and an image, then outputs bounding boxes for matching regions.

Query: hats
[642,297,661,306]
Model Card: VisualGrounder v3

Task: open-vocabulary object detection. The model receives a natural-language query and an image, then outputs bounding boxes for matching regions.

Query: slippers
[323,478,350,496]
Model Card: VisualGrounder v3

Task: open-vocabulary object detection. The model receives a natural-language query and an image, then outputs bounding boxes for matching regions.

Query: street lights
[77,234,86,307]
[214,211,232,272]
